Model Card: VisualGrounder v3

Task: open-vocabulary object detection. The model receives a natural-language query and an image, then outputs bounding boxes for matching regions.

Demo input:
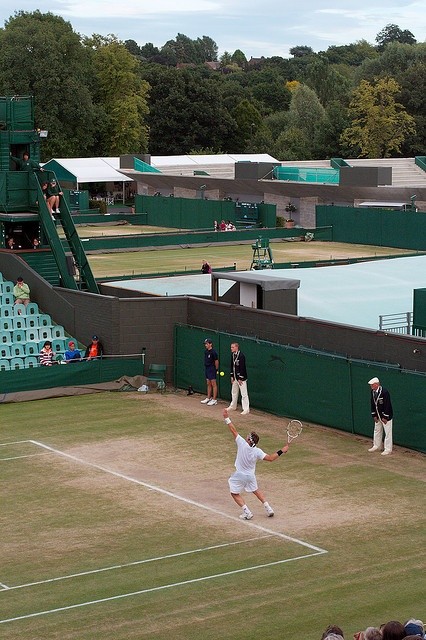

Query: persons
[40,341,57,366]
[13,277,31,305]
[231,223,237,231]
[202,258,212,274]
[7,238,19,249]
[379,618,425,639]
[222,409,288,521]
[217,219,226,231]
[368,377,393,456]
[48,178,63,213]
[83,334,102,360]
[224,342,250,414]
[200,339,219,406]
[34,236,45,248]
[226,221,232,231]
[36,182,56,220]
[64,340,83,364]
[322,621,382,639]
[9,151,44,171]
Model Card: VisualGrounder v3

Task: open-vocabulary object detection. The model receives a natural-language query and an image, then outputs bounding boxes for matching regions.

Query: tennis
[220,372,225,376]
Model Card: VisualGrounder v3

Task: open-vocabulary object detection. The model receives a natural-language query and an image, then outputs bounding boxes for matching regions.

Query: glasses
[93,339,97,341]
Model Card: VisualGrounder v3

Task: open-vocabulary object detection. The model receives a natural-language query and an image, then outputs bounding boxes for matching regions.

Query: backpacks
[205,265,212,273]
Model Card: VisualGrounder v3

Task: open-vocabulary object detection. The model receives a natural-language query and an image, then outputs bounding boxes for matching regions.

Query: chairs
[65,338,83,351]
[13,304,26,318]
[71,338,72,339]
[3,281,15,295]
[52,326,69,341]
[0,272,4,285]
[0,285,2,296]
[52,339,65,353]
[26,330,39,343]
[11,358,24,370]
[1,293,14,307]
[81,350,87,358]
[0,296,2,305]
[38,314,54,328]
[0,332,11,347]
[0,318,14,332]
[252,237,269,249]
[11,344,24,358]
[38,327,52,342]
[13,317,26,332]
[0,344,11,360]
[38,356,40,367]
[25,343,39,356]
[39,341,45,351]
[24,357,37,369]
[0,305,13,319]
[52,354,64,362]
[27,316,38,329]
[27,303,39,318]
[12,330,26,345]
[0,360,10,371]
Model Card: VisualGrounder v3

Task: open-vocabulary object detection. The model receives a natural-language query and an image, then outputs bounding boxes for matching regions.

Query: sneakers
[51,209,55,213]
[238,511,253,519]
[368,445,384,453]
[207,399,218,406]
[266,508,274,517]
[55,208,61,214]
[381,449,393,456]
[241,410,250,415]
[225,406,237,411]
[51,215,56,221]
[200,397,213,404]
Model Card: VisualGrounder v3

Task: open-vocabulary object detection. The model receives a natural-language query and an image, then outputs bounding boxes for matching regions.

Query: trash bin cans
[286,219,294,228]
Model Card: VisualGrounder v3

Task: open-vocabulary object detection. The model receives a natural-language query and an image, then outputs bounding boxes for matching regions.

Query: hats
[44,341,51,345]
[49,178,56,182]
[203,339,213,344]
[69,341,75,346]
[91,335,98,340]
[367,376,380,385]
[16,277,23,282]
